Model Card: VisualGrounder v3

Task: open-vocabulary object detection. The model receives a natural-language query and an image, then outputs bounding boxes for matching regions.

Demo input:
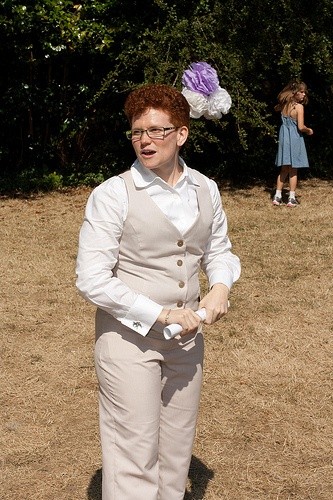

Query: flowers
[181,62,231,121]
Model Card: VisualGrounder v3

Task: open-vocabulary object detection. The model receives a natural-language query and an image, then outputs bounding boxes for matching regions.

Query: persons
[73,80,243,500]
[274,78,314,204]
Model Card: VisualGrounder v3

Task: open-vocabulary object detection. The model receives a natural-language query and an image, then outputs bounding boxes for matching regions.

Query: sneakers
[272,195,286,206]
[286,198,300,207]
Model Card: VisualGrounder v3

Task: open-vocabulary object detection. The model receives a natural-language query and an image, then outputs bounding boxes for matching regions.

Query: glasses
[123,127,179,141]
